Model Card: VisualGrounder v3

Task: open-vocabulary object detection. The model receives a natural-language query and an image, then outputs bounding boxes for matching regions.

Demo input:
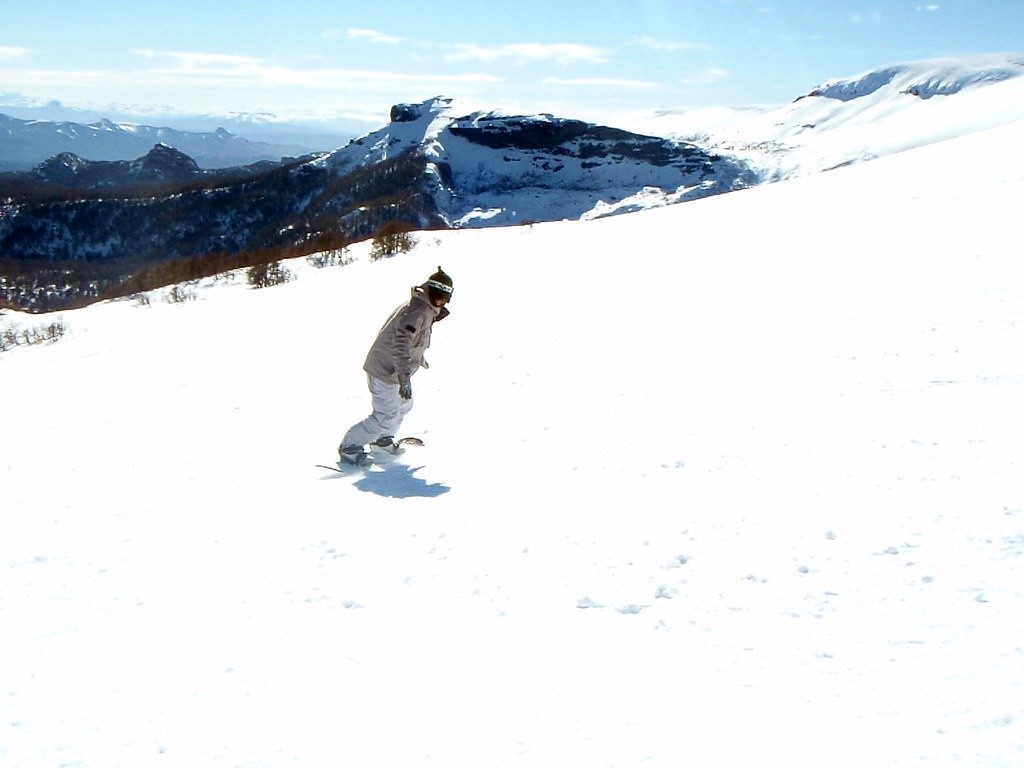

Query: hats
[428,266,454,303]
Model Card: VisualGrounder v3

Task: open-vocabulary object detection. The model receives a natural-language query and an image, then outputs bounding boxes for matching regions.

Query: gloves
[398,381,412,400]
[420,355,429,370]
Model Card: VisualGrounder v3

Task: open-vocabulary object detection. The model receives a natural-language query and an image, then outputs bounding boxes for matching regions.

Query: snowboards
[316,438,424,473]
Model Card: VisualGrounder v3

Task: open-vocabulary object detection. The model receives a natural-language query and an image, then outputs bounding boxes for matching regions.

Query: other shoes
[377,437,405,456]
[338,445,374,466]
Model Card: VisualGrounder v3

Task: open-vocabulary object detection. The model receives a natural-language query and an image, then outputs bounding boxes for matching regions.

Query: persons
[338,265,453,467]
